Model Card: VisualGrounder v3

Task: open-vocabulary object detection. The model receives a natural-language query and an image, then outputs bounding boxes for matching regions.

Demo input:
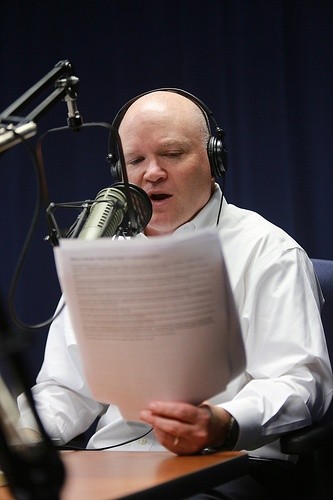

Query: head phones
[107,88,228,188]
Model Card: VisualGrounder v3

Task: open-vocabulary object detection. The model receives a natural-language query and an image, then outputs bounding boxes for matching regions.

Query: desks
[0,445,250,500]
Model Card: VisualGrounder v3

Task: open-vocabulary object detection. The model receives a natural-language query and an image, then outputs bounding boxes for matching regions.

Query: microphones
[77,187,127,239]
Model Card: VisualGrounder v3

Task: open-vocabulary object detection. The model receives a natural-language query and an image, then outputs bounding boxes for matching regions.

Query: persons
[17,88,333,452]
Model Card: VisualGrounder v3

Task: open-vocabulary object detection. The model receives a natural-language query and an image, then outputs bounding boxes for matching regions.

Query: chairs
[249,259,333,500]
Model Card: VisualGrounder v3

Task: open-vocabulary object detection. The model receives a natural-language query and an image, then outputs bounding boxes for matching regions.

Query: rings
[171,437,179,448]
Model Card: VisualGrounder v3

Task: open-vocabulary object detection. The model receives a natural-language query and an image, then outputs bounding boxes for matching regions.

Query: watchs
[228,416,240,448]
